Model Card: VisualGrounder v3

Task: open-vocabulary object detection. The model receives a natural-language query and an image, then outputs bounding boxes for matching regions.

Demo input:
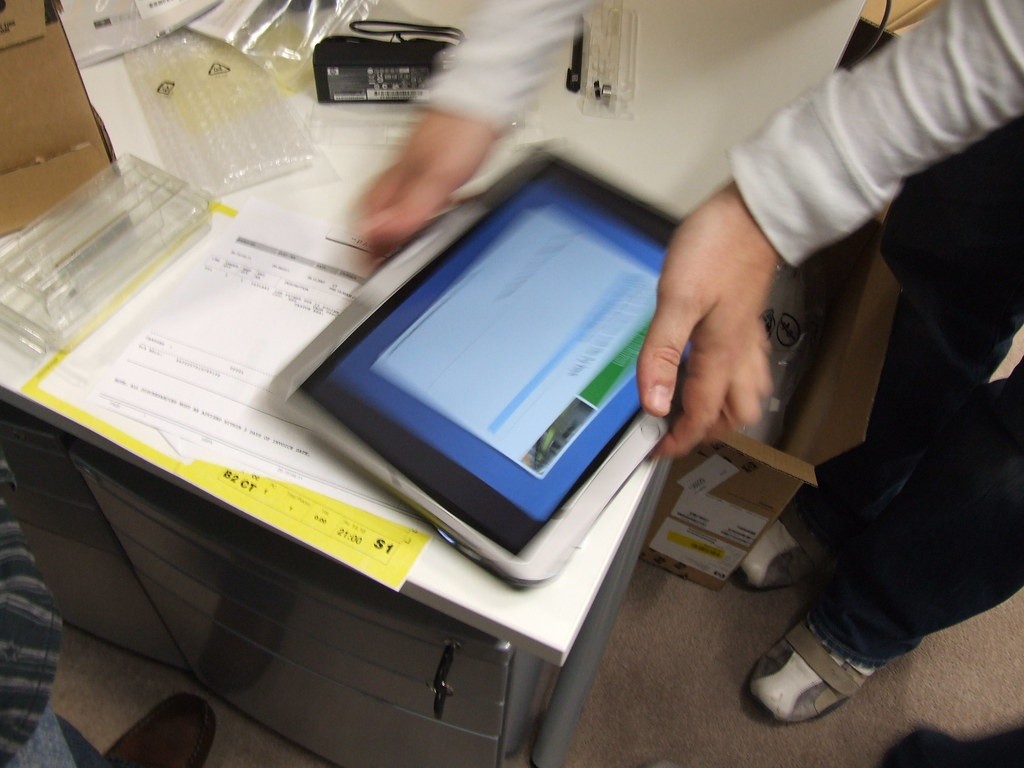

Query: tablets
[267,148,684,584]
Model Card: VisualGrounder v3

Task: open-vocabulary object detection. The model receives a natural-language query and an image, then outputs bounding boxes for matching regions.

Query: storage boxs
[0,0,124,240]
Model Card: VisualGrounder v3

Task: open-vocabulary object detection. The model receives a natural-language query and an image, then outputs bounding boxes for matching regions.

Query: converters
[313,39,456,102]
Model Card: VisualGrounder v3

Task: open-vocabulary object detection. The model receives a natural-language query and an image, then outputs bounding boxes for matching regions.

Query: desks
[0,0,868,768]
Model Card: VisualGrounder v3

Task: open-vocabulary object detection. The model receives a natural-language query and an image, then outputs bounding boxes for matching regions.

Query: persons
[0,458,215,768]
[352,2,1024,725]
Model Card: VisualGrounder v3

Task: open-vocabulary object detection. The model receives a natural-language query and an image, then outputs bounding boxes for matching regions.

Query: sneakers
[749,620,878,725]
[740,517,817,591]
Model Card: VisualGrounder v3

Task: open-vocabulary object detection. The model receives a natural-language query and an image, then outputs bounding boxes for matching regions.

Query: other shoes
[104,692,216,768]
